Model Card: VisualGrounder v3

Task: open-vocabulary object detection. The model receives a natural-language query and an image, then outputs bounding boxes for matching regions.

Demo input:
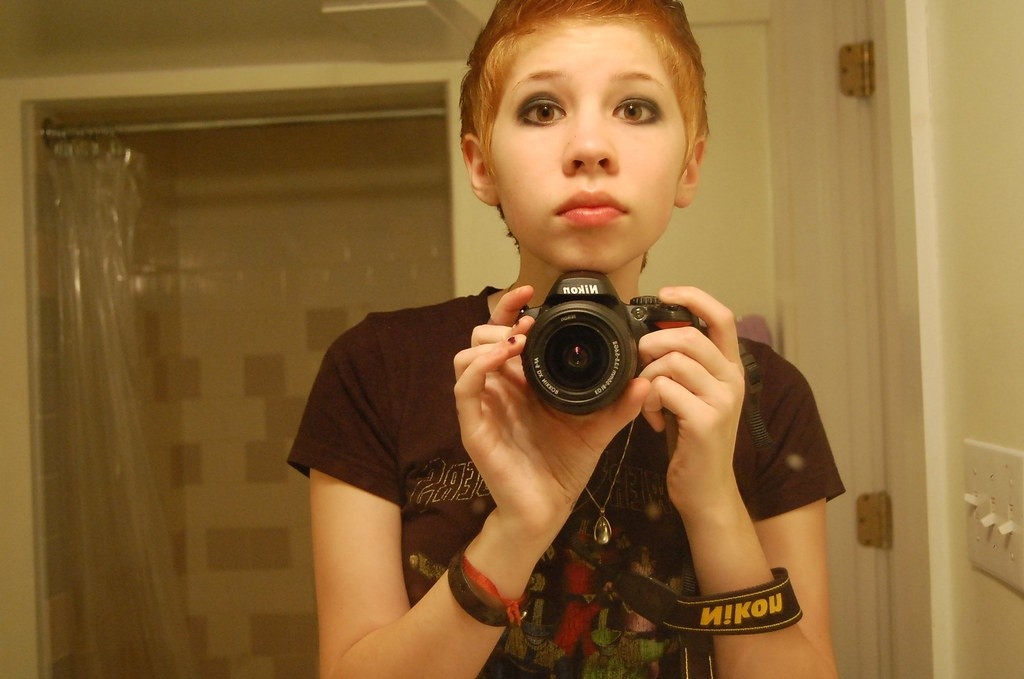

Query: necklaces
[585,419,636,545]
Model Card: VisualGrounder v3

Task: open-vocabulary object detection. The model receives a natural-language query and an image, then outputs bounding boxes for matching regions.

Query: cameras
[516,269,701,416]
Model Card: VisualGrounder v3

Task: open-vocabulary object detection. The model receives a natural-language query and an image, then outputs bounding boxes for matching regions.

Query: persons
[285,0,847,679]
